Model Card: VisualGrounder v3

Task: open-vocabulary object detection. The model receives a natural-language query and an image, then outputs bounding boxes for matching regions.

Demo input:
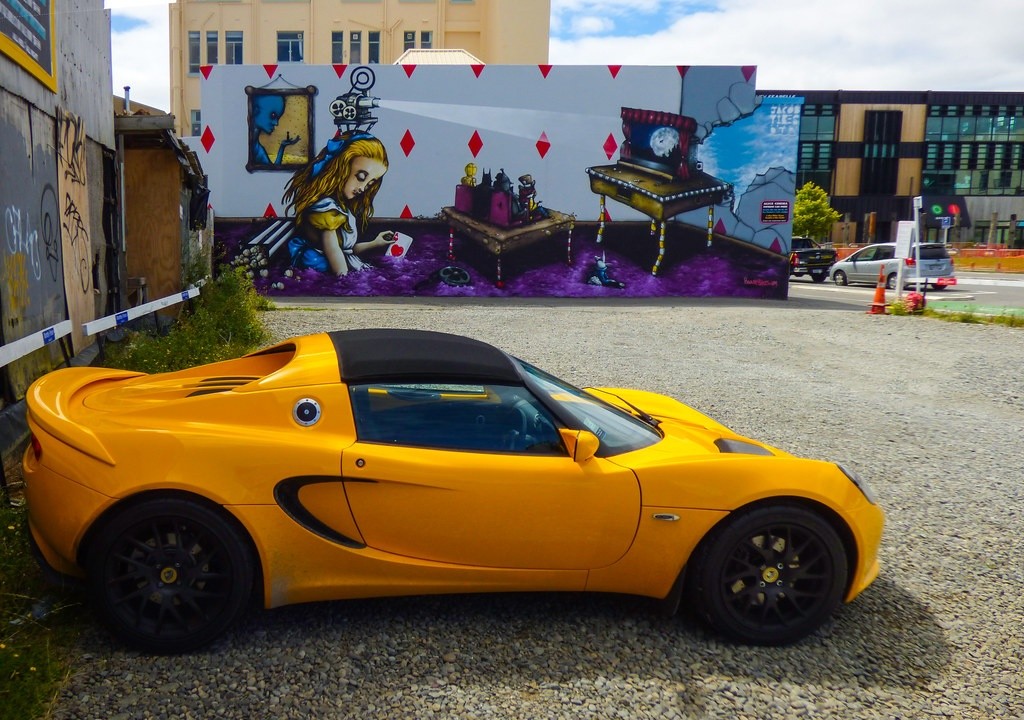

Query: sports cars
[21,328,887,655]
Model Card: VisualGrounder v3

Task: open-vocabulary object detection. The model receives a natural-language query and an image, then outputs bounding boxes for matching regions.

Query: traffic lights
[921,195,973,231]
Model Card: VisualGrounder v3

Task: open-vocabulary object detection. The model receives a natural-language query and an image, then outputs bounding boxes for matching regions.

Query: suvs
[829,240,957,290]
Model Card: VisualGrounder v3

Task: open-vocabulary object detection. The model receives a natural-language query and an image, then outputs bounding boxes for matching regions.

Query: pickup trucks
[791,235,838,283]
